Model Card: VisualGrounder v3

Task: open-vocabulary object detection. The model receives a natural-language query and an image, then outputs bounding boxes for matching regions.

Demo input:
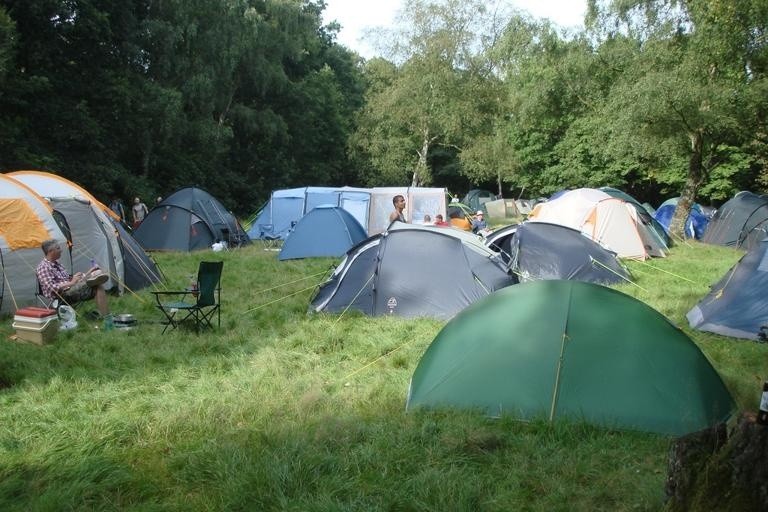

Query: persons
[434,215,449,226]
[421,214,432,225]
[390,195,408,224]
[450,194,460,203]
[36,238,117,331]
[472,210,489,240]
[132,196,149,227]
[109,195,127,222]
[154,196,162,204]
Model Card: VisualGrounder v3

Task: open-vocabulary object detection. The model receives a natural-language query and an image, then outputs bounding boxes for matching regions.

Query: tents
[462,190,497,210]
[257,189,447,236]
[406,279,738,438]
[130,185,253,252]
[1,168,166,317]
[482,198,522,220]
[309,226,520,320]
[685,237,768,342]
[279,206,368,259]
[655,198,707,239]
[534,188,670,262]
[602,186,676,258]
[515,198,536,215]
[481,222,636,285]
[701,190,768,251]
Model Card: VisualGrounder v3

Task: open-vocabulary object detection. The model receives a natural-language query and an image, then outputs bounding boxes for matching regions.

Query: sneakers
[87,274,108,287]
[104,314,115,322]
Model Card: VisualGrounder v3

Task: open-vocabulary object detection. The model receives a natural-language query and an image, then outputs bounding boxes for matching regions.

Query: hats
[477,210,484,216]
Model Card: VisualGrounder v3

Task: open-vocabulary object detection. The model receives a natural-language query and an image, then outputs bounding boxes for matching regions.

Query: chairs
[150,261,225,334]
[259,223,281,250]
[32,269,94,325]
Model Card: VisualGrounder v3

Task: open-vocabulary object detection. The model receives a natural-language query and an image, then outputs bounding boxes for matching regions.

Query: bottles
[190,276,199,297]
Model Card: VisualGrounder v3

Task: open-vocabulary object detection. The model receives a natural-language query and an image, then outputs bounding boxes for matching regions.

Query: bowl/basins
[118,313,132,322]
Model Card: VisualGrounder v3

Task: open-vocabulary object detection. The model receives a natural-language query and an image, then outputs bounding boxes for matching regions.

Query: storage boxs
[11,308,59,348]
